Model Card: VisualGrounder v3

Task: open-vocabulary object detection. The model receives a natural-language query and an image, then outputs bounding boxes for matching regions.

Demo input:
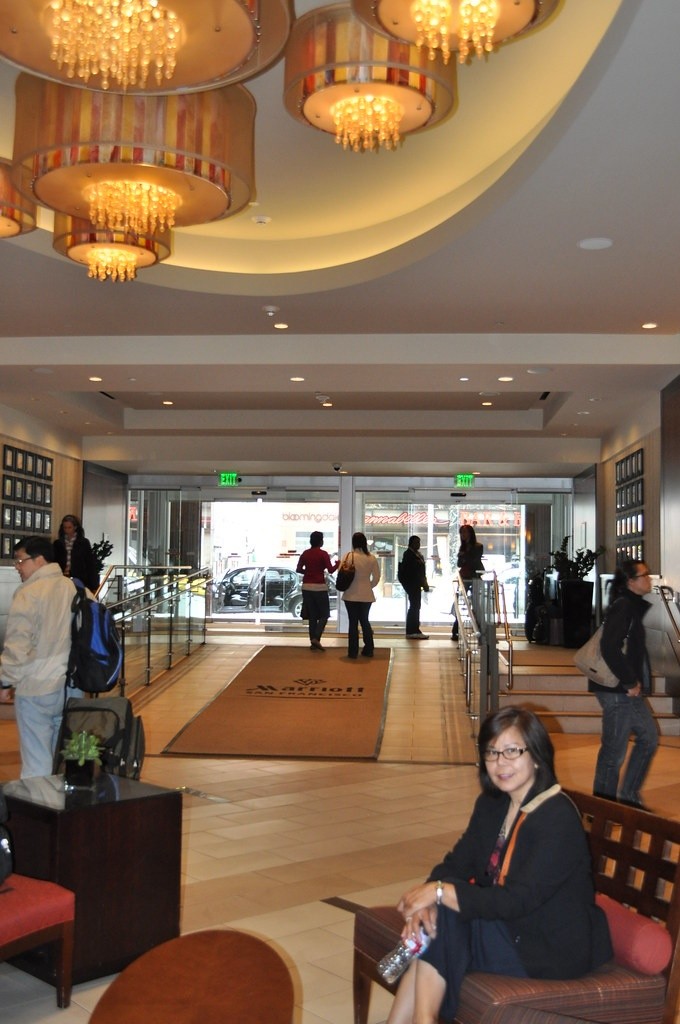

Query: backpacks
[51,695,145,780]
[66,578,124,694]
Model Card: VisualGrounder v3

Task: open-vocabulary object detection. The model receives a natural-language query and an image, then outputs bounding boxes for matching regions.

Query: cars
[213,565,339,620]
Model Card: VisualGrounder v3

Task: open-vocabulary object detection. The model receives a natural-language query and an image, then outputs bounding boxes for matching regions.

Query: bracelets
[436,881,442,904]
[0,684,12,689]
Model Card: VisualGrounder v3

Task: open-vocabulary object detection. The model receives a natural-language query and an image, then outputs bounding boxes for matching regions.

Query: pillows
[593,890,673,976]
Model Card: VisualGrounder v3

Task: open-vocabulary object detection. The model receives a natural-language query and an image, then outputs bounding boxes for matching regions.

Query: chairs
[86,926,304,1024]
[0,873,75,1008]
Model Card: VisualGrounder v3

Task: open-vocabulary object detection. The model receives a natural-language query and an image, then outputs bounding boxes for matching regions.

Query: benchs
[353,785,680,1024]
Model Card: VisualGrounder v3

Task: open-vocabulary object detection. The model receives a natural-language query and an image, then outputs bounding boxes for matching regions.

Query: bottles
[375,925,430,984]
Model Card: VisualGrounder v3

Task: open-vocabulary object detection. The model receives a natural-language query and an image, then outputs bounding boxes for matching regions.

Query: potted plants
[60,731,106,785]
[548,534,606,648]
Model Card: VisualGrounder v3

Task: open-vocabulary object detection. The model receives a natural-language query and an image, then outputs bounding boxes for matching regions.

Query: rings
[406,916,412,921]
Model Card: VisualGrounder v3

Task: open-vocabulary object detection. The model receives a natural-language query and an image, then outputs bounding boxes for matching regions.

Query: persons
[296,531,341,651]
[399,535,429,638]
[588,559,658,826]
[53,514,97,593]
[387,706,612,1024]
[450,524,485,641]
[0,536,82,779]
[338,532,380,658]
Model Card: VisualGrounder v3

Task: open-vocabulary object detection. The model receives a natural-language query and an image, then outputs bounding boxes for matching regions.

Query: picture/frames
[614,449,645,566]
[0,444,54,559]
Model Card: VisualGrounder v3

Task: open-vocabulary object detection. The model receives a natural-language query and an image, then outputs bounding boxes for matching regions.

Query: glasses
[629,570,650,577]
[484,747,532,762]
[13,556,37,566]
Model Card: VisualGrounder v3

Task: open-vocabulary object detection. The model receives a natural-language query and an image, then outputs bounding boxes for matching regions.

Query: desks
[3,773,188,988]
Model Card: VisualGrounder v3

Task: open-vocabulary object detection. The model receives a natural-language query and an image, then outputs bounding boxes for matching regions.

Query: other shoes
[451,633,458,640]
[310,639,325,650]
[588,812,623,827]
[406,631,429,639]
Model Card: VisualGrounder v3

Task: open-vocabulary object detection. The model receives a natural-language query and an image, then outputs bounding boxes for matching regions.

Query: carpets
[161,643,393,760]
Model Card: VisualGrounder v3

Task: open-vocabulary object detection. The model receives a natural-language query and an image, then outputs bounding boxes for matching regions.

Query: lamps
[0,0,563,283]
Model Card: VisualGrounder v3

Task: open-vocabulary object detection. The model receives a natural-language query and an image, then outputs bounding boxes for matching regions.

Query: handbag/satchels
[335,552,355,592]
[573,596,635,688]
